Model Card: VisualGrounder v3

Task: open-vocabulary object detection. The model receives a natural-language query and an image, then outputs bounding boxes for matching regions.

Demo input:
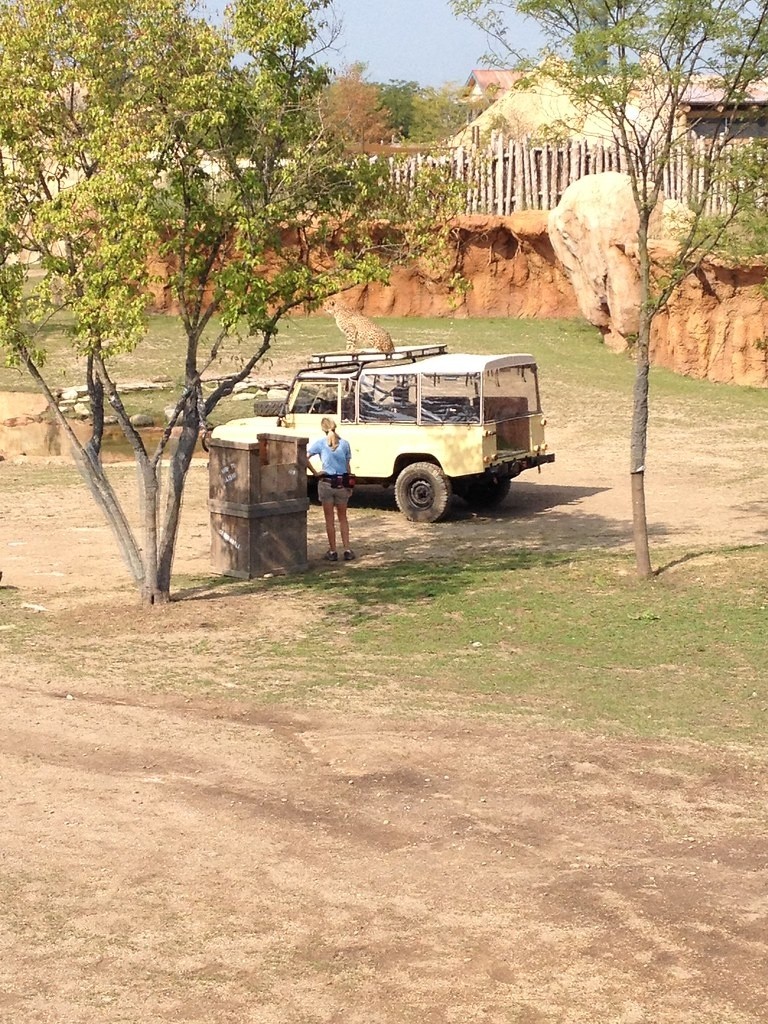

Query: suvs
[212,343,555,522]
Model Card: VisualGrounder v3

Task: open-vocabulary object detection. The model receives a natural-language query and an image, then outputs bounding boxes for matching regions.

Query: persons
[305,417,356,561]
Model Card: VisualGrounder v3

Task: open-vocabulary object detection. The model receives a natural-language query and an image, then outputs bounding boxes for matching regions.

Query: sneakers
[323,549,338,560]
[344,550,355,560]
